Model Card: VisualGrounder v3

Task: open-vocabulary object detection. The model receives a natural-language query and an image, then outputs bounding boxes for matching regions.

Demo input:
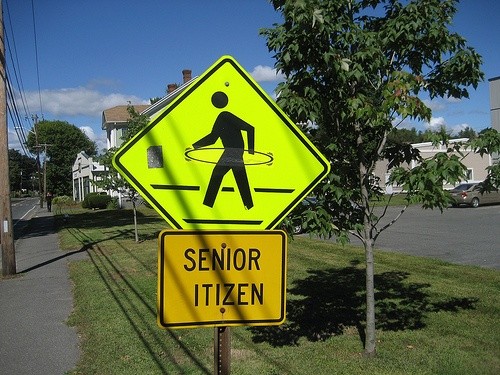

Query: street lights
[14,127,41,205]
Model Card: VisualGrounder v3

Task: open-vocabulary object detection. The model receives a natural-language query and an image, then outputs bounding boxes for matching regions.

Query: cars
[446,183,500,208]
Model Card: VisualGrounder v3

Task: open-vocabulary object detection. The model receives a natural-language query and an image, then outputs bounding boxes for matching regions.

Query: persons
[45,192,52,212]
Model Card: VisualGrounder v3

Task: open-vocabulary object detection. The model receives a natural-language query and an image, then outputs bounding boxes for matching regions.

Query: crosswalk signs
[111,55,331,329]
